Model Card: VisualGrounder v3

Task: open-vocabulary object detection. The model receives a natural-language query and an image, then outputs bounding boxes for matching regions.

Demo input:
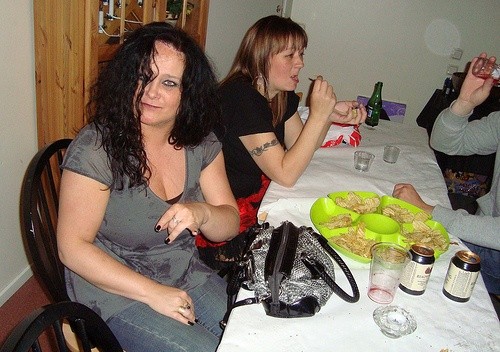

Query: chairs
[0,301,124,352]
[18,139,126,352]
[354,95,407,124]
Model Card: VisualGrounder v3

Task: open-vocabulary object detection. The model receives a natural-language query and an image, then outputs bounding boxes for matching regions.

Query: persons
[195,14,367,266]
[57,21,241,352]
[392,53,500,297]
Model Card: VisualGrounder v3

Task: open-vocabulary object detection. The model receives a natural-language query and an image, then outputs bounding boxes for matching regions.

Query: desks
[215,116,500,352]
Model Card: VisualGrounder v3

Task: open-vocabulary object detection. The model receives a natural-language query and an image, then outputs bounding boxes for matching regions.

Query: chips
[318,213,352,229]
[328,222,408,263]
[382,202,429,224]
[400,219,449,251]
[335,191,380,215]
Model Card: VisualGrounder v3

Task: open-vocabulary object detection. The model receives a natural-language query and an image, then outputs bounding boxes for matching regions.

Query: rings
[178,301,191,313]
[173,217,179,224]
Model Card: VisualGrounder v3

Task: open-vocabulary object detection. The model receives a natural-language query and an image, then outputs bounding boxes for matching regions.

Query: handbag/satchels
[226,221,360,319]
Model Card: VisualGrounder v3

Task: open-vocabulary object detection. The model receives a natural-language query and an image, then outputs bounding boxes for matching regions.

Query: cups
[382,145,401,164]
[354,150,375,174]
[472,57,500,88]
[367,242,413,304]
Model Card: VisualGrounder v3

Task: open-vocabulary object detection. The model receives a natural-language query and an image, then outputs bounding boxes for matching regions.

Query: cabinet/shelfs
[33,0,210,267]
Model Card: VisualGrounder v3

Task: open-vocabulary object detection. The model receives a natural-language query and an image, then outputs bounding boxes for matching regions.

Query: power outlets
[446,63,459,75]
[450,47,464,60]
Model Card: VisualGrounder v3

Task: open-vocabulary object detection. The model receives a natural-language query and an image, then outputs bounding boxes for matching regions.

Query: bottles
[365,81,383,126]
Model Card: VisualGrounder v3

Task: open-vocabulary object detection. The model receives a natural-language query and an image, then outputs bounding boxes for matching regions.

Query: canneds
[399,243,435,296]
[443,250,482,303]
